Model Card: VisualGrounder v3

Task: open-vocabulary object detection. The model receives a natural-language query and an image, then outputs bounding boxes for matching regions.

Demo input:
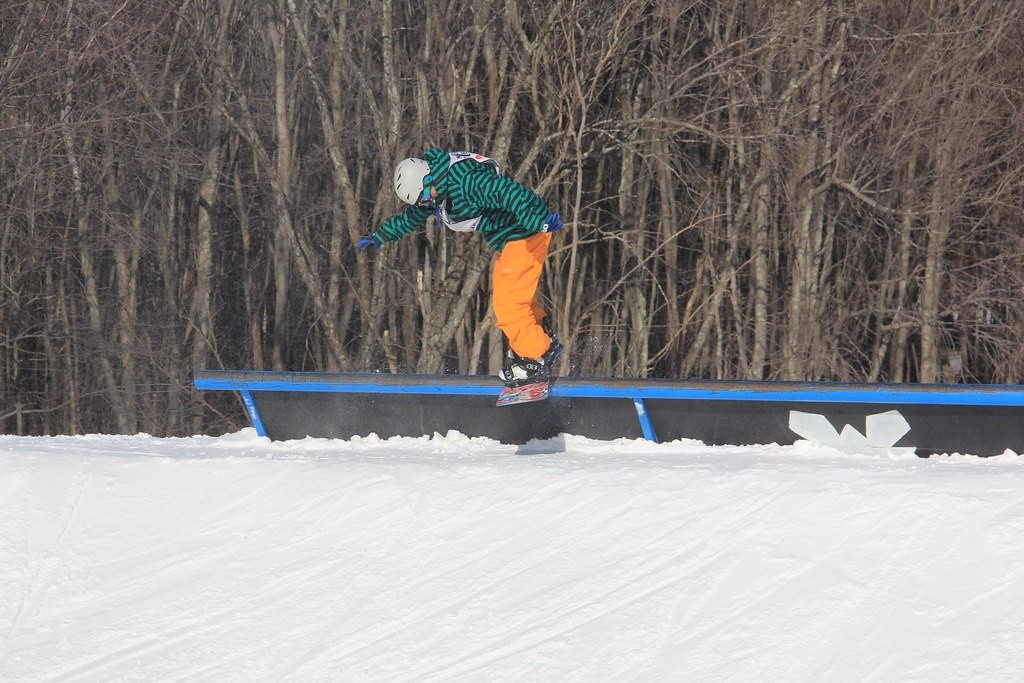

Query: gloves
[542,213,563,234]
[358,234,382,250]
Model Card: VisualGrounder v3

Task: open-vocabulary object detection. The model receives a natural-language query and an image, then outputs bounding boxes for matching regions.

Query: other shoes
[499,333,564,384]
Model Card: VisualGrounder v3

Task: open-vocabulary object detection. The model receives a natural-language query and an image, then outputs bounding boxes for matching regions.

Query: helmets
[393,159,430,205]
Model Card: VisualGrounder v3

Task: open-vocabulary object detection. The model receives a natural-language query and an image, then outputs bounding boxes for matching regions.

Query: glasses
[418,195,431,206]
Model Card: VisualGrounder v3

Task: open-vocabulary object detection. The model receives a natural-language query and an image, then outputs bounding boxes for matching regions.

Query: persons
[358,148,564,387]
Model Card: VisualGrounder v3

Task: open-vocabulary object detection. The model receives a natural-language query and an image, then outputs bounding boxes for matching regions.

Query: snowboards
[494,360,549,407]
